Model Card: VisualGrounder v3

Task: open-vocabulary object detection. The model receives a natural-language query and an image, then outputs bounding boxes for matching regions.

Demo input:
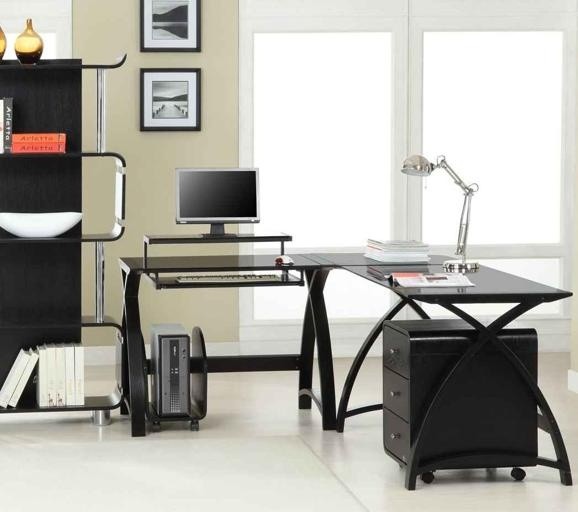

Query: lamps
[400,153,481,272]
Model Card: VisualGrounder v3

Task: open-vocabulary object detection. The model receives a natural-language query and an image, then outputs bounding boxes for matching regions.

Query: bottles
[0,18,44,65]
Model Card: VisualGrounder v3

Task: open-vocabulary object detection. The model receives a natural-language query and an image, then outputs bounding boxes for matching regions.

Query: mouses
[273,255,295,265]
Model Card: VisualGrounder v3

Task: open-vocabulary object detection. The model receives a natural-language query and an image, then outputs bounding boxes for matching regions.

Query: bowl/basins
[0,212,85,238]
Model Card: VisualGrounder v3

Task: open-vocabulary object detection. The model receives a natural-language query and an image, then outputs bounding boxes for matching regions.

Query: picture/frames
[139,0,202,132]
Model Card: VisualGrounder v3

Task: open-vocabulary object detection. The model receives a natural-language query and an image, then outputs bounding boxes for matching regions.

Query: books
[386,271,476,288]
[0,341,85,410]
[366,264,430,281]
[363,236,433,263]
[0,96,67,153]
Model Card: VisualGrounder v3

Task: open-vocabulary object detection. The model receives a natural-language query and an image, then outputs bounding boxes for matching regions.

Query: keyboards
[174,274,282,283]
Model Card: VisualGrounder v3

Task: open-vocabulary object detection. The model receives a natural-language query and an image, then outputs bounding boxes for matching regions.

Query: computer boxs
[150,323,192,417]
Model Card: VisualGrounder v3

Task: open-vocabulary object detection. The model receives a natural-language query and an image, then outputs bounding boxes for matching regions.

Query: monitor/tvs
[174,166,261,238]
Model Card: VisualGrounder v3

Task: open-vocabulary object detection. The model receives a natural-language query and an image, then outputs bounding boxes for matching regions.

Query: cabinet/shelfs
[0,52,130,428]
[382,318,539,483]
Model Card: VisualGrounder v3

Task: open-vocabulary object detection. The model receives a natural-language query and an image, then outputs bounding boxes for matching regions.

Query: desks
[115,251,574,491]
